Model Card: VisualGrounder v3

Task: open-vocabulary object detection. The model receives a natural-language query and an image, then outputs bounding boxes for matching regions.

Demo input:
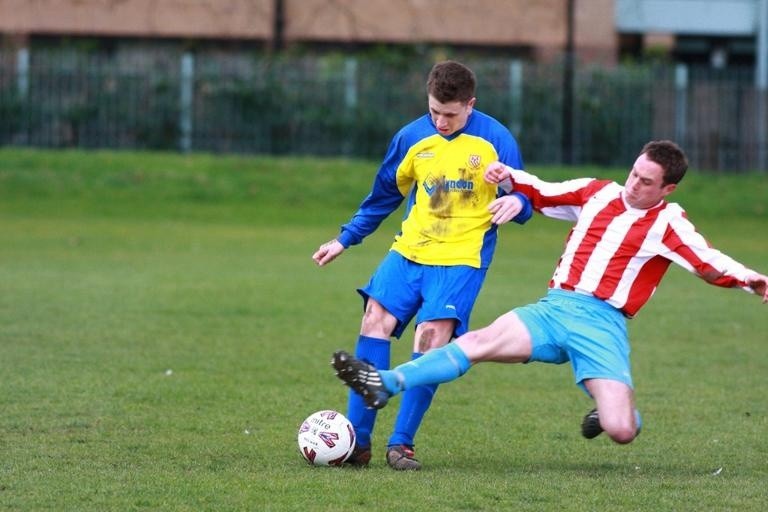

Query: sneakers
[344,443,372,466]
[580,409,604,439]
[330,350,390,410]
[386,443,422,470]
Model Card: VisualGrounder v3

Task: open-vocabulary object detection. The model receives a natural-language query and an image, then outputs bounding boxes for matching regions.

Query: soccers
[298,410,357,467]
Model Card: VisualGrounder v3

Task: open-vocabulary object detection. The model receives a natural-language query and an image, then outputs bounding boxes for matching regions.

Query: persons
[311,60,533,471]
[330,140,768,444]
